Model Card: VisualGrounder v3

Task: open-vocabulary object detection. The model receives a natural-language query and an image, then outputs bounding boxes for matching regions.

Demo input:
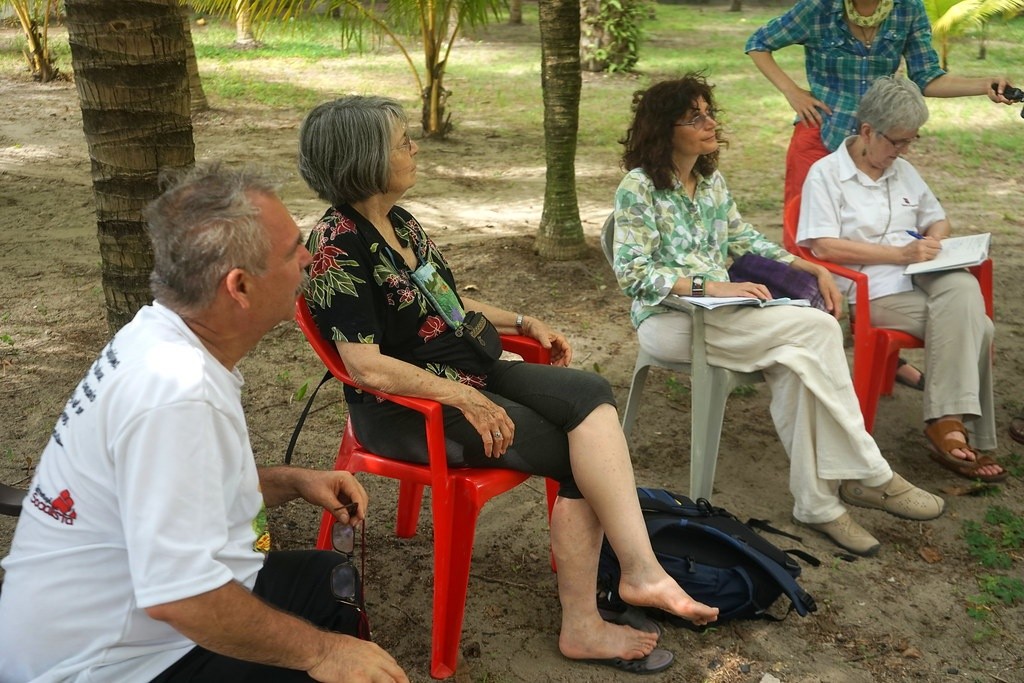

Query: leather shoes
[1008,417,1024,445]
[838,468,946,522]
[790,501,880,557]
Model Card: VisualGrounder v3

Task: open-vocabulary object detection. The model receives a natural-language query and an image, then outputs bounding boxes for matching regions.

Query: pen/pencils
[906,230,923,239]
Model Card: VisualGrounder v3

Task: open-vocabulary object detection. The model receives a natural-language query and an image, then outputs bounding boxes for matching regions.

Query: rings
[495,432,500,437]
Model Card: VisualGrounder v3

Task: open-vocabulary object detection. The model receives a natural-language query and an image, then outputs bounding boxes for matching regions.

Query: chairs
[297,291,559,679]
[784,196,992,440]
[603,209,767,508]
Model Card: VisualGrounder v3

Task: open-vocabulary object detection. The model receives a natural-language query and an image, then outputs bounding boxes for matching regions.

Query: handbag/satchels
[416,309,503,376]
[727,250,831,314]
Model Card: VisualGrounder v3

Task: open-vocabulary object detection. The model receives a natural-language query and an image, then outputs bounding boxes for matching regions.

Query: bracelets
[516,314,524,335]
[690,276,706,296]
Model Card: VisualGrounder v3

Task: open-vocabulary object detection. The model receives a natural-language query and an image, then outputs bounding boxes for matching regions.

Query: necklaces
[860,26,876,49]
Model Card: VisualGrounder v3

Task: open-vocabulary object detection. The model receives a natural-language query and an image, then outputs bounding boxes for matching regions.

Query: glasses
[873,127,921,149]
[670,104,716,130]
[390,131,413,151]
[329,502,366,609]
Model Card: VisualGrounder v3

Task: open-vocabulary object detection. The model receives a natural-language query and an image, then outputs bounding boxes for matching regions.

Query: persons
[796,75,1009,481]
[296,94,719,659]
[0,169,408,683]
[744,0,1013,258]
[613,78,946,554]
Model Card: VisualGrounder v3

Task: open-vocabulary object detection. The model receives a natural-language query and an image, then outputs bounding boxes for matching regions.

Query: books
[681,297,811,310]
[904,232,992,274]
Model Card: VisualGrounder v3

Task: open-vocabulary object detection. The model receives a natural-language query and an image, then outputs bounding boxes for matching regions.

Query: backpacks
[588,487,821,627]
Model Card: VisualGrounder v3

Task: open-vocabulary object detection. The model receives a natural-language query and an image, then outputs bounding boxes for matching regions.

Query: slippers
[595,605,661,642]
[575,648,675,675]
[923,417,979,467]
[933,448,1009,482]
